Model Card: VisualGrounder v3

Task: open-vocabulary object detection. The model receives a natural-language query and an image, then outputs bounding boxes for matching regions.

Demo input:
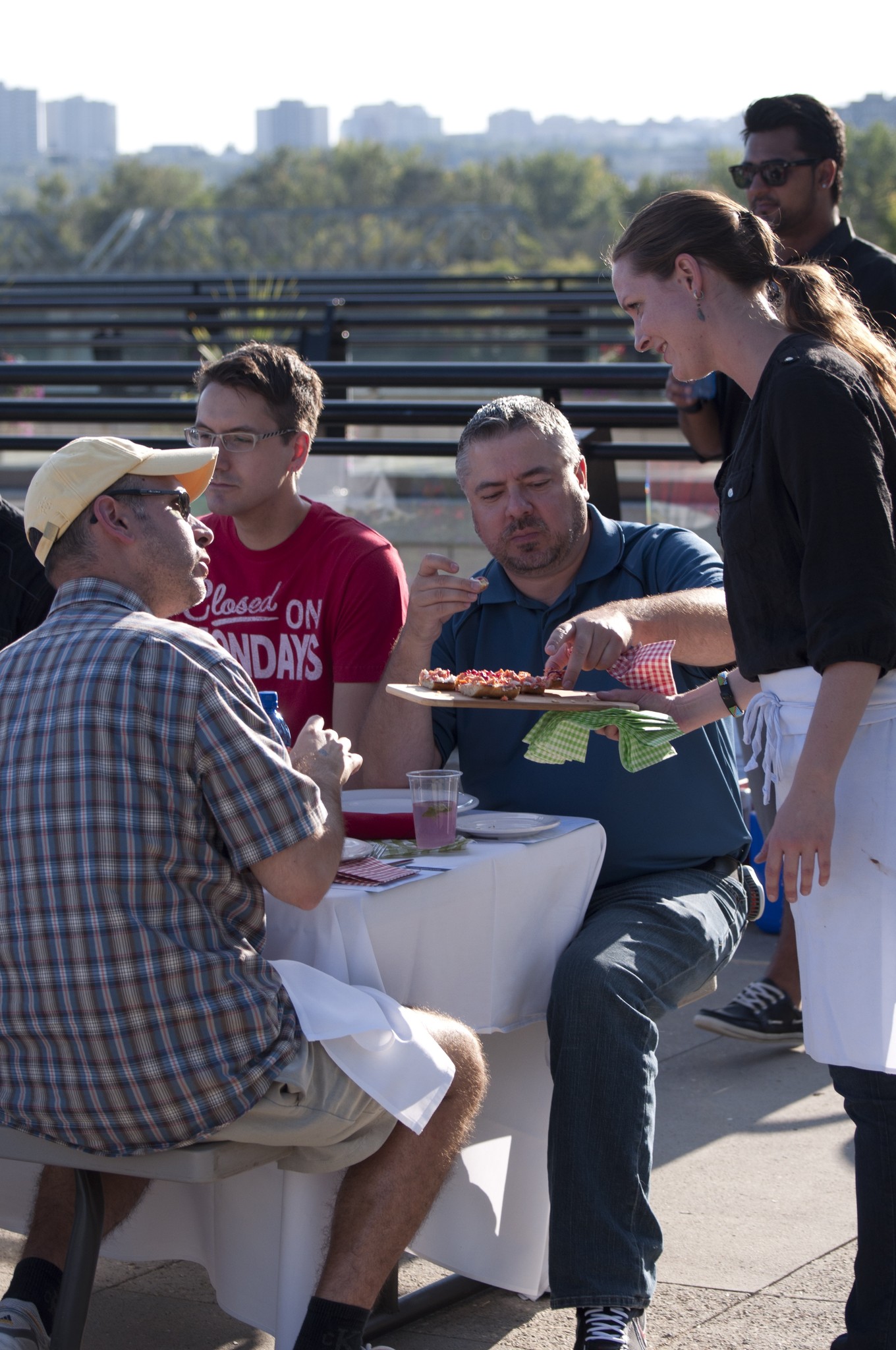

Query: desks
[0,787,610,1350]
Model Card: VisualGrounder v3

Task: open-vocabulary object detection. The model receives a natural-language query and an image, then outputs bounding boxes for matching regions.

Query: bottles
[257,690,291,758]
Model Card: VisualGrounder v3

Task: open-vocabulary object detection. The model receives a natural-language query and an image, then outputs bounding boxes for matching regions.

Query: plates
[341,787,478,818]
[456,813,560,839]
[339,835,374,865]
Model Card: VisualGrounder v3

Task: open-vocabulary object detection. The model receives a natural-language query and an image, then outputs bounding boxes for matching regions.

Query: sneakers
[693,977,804,1045]
[573,1305,647,1350]
[0,1298,51,1350]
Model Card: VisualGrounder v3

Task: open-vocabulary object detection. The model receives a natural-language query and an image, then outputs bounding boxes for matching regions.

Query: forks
[363,842,391,861]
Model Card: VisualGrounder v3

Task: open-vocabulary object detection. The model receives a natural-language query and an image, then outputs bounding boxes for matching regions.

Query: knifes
[386,858,415,867]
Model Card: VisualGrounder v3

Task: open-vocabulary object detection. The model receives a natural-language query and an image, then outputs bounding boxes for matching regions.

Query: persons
[356,395,755,1350]
[663,91,896,1046]
[165,338,414,790]
[589,188,896,1350]
[0,433,492,1350]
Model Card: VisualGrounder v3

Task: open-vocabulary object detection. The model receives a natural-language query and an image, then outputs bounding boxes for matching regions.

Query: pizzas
[417,667,568,698]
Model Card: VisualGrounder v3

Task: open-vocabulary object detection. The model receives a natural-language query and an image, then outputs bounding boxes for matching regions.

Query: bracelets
[674,395,707,415]
[718,671,745,719]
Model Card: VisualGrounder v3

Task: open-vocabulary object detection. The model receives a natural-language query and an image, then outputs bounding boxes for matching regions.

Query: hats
[24,436,219,566]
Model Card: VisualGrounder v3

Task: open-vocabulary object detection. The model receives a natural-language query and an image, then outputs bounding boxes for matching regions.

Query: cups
[406,768,464,851]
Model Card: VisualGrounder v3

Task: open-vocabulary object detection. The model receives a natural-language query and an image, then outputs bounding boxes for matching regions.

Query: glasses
[90,489,191,524]
[728,157,821,189]
[184,427,298,452]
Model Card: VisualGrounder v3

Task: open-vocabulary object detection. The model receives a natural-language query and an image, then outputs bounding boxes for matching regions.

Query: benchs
[0,1120,293,1350]
